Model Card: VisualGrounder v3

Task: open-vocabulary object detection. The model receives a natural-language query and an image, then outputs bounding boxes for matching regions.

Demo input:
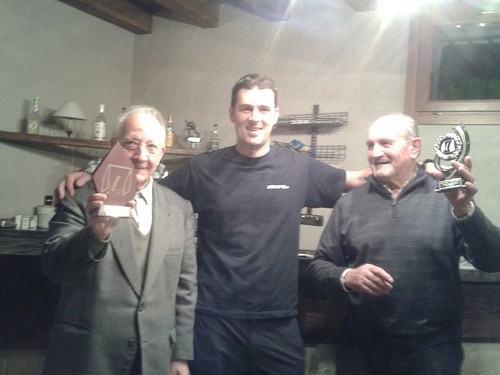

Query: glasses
[115,139,163,155]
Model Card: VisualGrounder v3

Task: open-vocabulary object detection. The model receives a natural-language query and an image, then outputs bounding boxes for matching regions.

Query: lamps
[51,99,88,137]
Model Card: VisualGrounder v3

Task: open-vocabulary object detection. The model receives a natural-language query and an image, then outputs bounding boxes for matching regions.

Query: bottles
[208,124,219,150]
[15,214,37,230]
[94,104,106,142]
[26,97,40,134]
[166,114,176,148]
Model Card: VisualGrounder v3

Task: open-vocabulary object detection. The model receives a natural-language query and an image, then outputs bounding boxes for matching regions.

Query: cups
[37,204,57,229]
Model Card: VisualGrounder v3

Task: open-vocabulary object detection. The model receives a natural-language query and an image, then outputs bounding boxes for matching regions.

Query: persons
[52,73,451,375]
[41,104,201,375]
[306,112,500,375]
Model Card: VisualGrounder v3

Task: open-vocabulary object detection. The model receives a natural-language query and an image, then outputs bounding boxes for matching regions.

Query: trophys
[91,141,138,218]
[435,121,471,193]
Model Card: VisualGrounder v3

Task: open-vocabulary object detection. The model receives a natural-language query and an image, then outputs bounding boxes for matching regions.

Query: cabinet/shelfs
[0,130,208,239]
[274,112,349,165]
